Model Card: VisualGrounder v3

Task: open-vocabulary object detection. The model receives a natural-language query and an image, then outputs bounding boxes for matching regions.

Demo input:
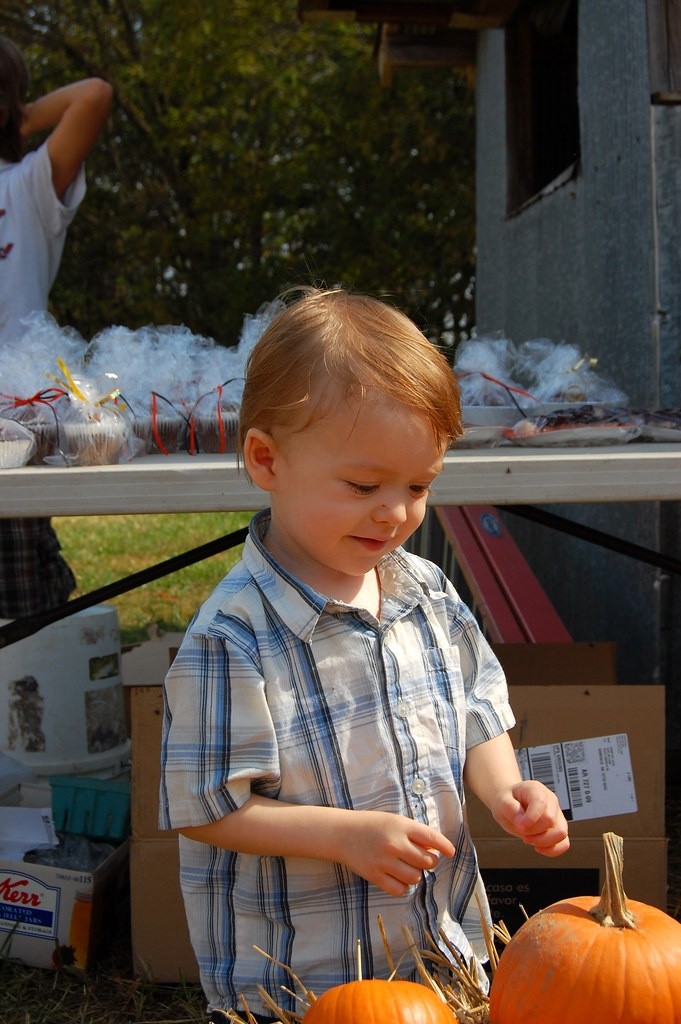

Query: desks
[0,443,681,650]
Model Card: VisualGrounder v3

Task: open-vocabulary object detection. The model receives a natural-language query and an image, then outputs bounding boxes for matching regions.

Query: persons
[161,291,570,1024]
[0,78,113,623]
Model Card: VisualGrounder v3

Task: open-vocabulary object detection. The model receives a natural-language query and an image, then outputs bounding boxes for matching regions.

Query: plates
[453,426,503,443]
[511,427,638,447]
[637,424,681,442]
[537,401,624,416]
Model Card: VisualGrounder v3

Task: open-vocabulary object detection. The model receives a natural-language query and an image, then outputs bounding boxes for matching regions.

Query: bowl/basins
[460,406,527,427]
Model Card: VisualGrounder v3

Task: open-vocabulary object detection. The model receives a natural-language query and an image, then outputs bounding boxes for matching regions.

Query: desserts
[0,391,247,469]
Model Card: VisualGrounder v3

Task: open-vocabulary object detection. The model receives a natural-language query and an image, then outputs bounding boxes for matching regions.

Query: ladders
[407,500,578,647]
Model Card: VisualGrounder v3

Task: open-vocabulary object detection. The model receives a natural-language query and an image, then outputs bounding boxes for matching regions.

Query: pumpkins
[302,979,458,1024]
[486,832,681,1024]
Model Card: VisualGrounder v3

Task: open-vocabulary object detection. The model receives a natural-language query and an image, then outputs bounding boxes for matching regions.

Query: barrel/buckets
[1,604,130,775]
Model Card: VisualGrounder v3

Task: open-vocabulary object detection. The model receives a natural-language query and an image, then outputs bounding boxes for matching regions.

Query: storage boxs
[49,772,130,843]
[0,841,130,973]
[133,641,671,984]
[0,783,59,845]
